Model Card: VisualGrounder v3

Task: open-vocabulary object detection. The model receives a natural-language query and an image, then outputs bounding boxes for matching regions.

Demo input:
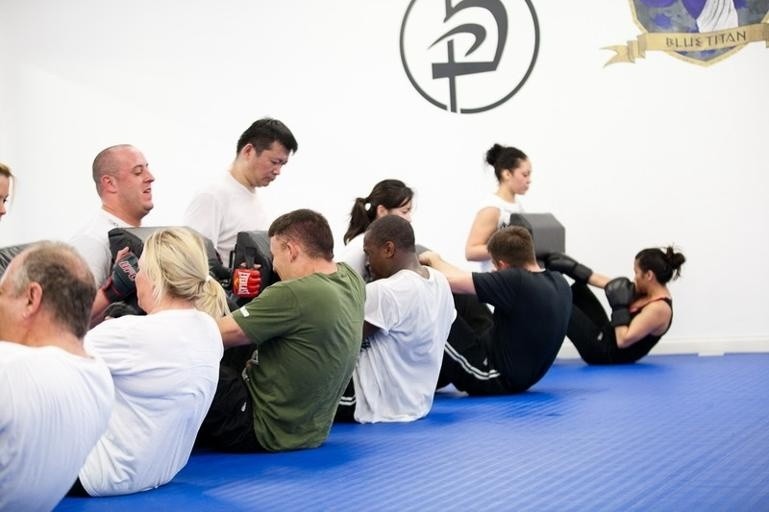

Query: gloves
[604,277,635,327]
[103,252,139,302]
[230,261,261,298]
[545,253,591,284]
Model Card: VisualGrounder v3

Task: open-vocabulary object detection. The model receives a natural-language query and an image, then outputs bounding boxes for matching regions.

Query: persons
[192,209,367,455]
[341,179,416,282]
[1,241,118,512]
[464,142,545,314]
[63,226,235,498]
[183,116,299,311]
[330,214,459,426]
[544,246,686,366]
[61,142,227,330]
[1,163,17,217]
[418,224,574,398]
